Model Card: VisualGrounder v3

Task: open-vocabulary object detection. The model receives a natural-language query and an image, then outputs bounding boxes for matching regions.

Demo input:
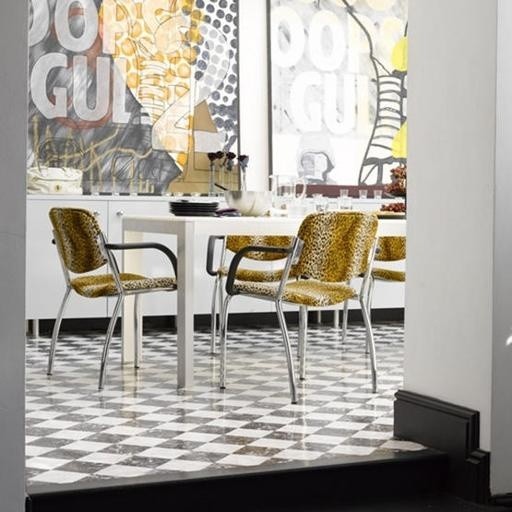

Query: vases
[208,162,216,196]
[228,165,234,189]
[218,165,226,195]
[240,170,248,191]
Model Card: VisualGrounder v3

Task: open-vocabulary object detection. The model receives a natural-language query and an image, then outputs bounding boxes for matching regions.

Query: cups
[339,188,349,197]
[314,196,329,213]
[374,190,382,199]
[339,196,353,210]
[359,189,368,200]
[268,173,307,216]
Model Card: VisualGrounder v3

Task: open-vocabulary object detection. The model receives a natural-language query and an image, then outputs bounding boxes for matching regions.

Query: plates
[169,201,218,217]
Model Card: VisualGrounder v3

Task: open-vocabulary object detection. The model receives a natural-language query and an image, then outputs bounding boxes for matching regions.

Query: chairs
[46,206,178,391]
[218,211,379,406]
[206,234,301,360]
[339,236,406,352]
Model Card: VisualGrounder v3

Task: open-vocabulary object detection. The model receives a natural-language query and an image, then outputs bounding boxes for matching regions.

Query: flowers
[225,152,236,160]
[207,153,217,162]
[217,151,224,160]
[236,155,250,164]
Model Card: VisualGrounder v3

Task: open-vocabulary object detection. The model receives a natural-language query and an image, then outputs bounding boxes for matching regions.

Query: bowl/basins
[224,190,273,217]
